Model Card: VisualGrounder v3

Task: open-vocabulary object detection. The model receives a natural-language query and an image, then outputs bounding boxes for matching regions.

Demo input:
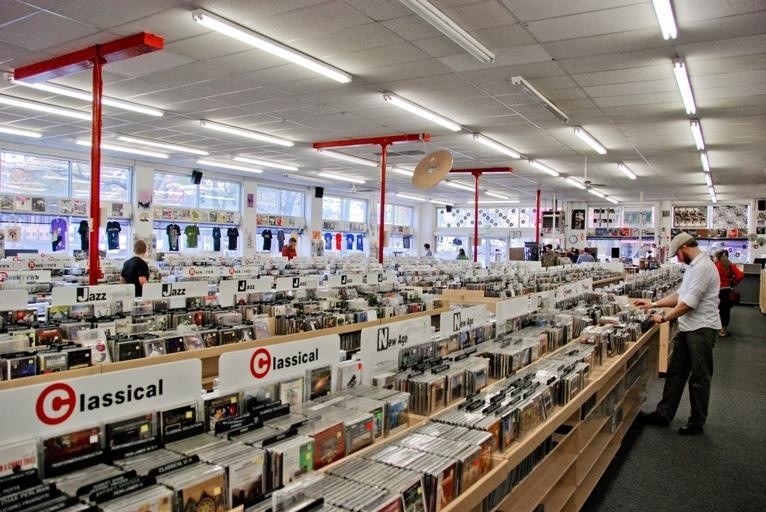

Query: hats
[668,232,694,258]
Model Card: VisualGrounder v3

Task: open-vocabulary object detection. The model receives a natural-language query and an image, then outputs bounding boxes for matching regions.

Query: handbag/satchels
[731,290,740,307]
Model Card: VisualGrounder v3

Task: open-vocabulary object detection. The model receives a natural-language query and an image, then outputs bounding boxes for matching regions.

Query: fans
[578,156,606,191]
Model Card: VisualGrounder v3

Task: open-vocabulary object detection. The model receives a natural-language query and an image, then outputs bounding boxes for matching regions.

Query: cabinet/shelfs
[0,199,766,511]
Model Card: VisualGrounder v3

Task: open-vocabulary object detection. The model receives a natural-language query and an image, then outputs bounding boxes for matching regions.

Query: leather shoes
[645,412,669,428]
[680,424,704,435]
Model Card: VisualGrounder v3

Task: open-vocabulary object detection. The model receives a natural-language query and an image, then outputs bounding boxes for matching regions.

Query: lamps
[511,74,567,121]
[686,120,719,197]
[530,157,557,178]
[572,125,607,157]
[673,59,703,117]
[473,134,518,155]
[1,70,476,198]
[565,177,604,197]
[191,7,352,87]
[653,0,679,45]
[401,0,495,65]
[617,162,637,181]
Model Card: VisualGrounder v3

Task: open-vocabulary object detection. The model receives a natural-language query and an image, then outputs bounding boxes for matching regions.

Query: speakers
[447,206,451,212]
[191,170,202,184]
[316,187,323,197]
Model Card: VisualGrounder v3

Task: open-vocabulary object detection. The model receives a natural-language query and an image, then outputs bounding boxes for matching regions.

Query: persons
[118,240,151,296]
[280,238,304,255]
[633,230,744,435]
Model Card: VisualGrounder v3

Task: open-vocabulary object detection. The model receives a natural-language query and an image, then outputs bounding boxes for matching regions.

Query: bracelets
[660,316,666,323]
